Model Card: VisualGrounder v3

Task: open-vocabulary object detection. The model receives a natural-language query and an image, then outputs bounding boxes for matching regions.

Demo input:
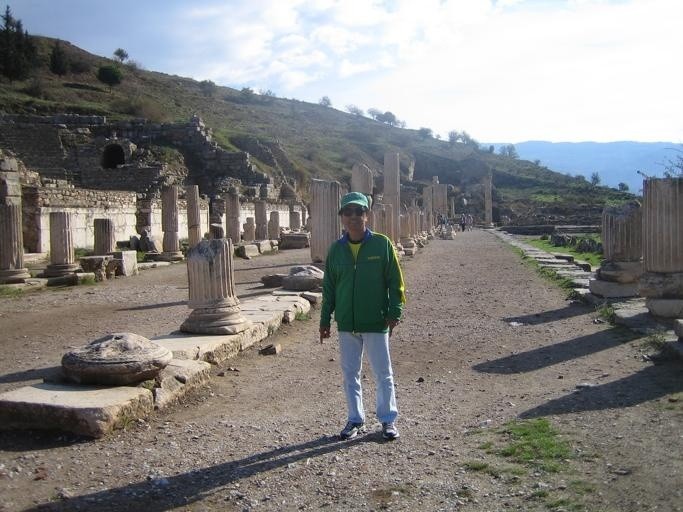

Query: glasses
[343,208,366,216]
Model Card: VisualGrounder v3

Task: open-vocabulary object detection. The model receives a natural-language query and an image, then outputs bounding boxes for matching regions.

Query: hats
[341,192,368,209]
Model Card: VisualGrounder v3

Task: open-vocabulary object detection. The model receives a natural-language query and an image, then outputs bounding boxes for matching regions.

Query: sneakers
[341,421,366,439]
[382,422,400,438]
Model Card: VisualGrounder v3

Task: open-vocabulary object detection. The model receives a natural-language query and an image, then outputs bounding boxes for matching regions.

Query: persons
[317,191,407,442]
[435,210,476,234]
